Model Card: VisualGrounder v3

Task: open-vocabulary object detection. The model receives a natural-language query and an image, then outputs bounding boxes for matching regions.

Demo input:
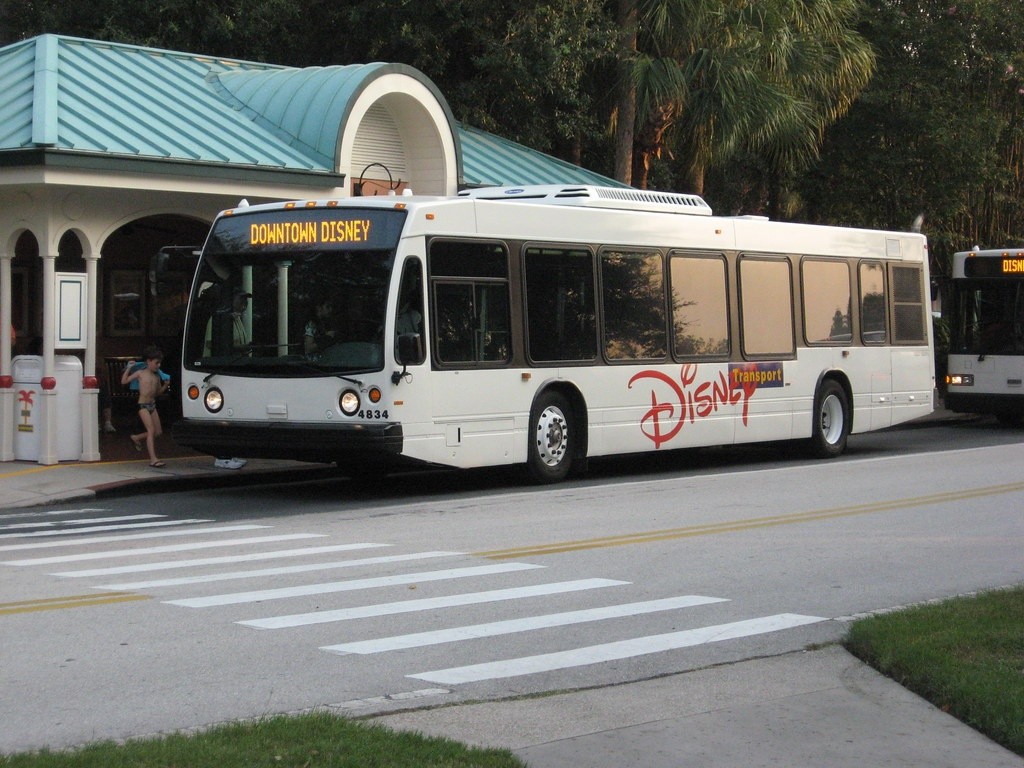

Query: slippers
[129,434,143,452]
[149,460,167,468]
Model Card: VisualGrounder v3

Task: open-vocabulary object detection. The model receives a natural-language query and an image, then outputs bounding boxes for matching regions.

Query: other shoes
[98,424,102,432]
[103,425,116,434]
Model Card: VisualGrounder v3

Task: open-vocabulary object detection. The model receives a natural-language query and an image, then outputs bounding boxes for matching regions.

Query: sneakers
[230,457,247,466]
[214,458,242,469]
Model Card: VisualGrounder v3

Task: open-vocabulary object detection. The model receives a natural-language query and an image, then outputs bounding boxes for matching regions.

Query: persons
[201,285,253,470]
[375,295,423,364]
[300,299,341,359]
[121,346,171,467]
[96,355,117,432]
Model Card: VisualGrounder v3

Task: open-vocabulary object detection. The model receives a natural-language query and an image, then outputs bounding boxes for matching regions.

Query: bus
[931,246,1024,426]
[149,183,940,483]
